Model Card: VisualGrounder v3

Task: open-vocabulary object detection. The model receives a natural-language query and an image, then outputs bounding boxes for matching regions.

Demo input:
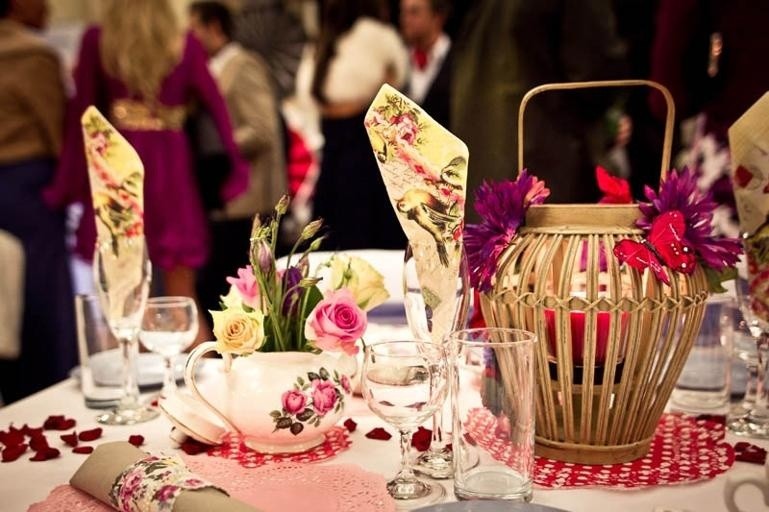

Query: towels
[724,94,765,330]
[82,106,144,318]
[364,83,470,344]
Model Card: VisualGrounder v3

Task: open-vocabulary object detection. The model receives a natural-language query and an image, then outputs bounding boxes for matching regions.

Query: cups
[73,294,127,407]
[665,297,734,415]
[451,328,539,504]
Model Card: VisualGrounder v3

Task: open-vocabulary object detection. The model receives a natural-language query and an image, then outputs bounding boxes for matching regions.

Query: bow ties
[410,49,427,69]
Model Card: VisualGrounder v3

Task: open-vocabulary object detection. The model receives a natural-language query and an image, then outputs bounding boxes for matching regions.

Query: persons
[181,3,290,327]
[309,1,412,249]
[0,1,68,402]
[43,0,254,358]
[396,1,451,138]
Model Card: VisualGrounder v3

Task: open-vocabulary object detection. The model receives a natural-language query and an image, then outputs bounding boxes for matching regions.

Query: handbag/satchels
[185,104,231,206]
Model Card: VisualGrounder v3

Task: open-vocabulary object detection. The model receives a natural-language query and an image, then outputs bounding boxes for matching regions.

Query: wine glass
[361,339,449,509]
[403,242,481,480]
[93,236,160,424]
[139,297,198,413]
[718,302,769,439]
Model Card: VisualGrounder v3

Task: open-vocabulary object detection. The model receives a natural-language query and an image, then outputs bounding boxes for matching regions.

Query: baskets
[478,79,709,466]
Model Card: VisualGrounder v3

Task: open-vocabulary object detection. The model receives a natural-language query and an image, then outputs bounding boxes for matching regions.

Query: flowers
[458,174,575,295]
[610,176,748,282]
[193,199,401,351]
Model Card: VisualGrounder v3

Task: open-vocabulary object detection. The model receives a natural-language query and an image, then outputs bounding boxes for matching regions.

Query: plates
[68,348,201,388]
